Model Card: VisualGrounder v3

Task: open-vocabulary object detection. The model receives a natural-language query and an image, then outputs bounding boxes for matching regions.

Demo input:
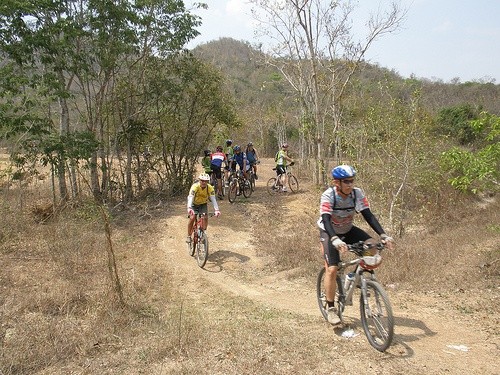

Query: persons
[202,149,213,174]
[245,142,260,179]
[317,166,393,325]
[225,140,234,171]
[210,146,229,198]
[186,173,221,244]
[229,145,250,196]
[276,144,294,192]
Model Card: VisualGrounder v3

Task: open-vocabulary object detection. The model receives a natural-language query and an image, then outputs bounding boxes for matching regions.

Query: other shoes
[186,237,192,243]
[218,194,224,199]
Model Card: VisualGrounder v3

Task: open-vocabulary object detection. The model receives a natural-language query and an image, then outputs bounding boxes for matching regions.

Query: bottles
[344,272,354,291]
[198,228,200,236]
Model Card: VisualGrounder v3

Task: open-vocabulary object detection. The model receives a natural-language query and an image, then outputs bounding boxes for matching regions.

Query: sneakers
[356,284,372,289]
[327,307,341,324]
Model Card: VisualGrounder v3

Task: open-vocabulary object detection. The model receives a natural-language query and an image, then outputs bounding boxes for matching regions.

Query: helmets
[204,150,212,154]
[217,146,223,151]
[233,145,241,150]
[247,142,253,146]
[199,173,210,180]
[332,164,356,178]
[226,139,232,145]
[282,144,289,148]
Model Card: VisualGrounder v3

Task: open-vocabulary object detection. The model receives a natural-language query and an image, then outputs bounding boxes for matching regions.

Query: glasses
[339,179,355,184]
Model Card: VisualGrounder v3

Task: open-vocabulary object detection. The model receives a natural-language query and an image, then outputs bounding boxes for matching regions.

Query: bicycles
[186,206,220,269]
[267,163,299,197]
[202,159,261,204]
[316,236,395,352]
